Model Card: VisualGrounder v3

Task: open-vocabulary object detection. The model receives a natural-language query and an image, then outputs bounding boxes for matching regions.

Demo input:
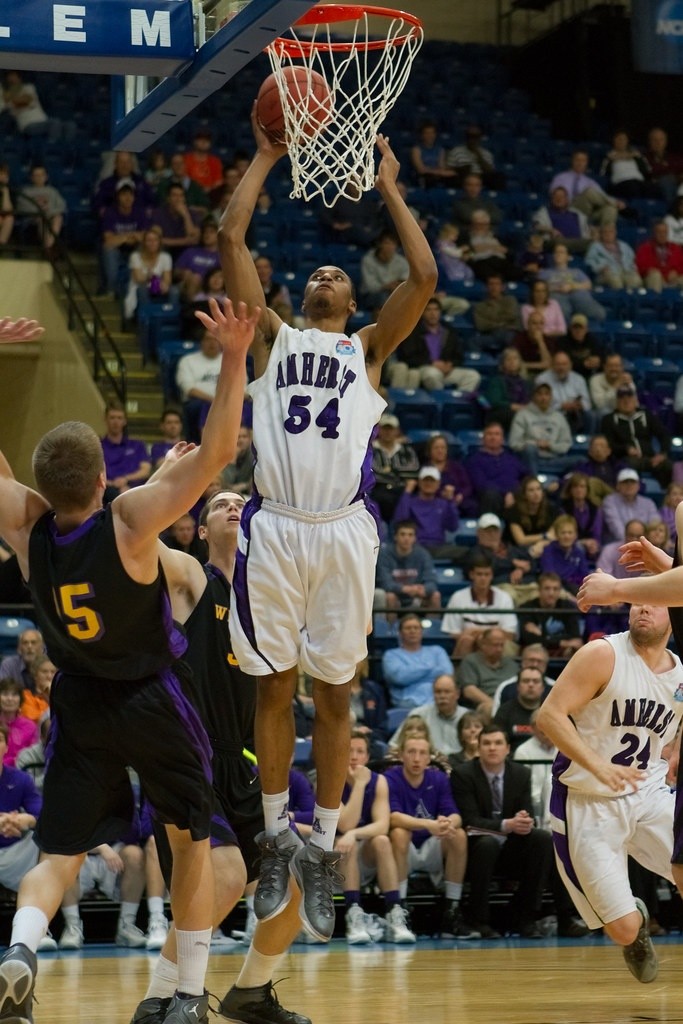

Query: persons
[536,572,683,983]
[576,498,683,904]
[128,437,314,1024]
[216,98,439,944]
[0,56,683,953]
[0,297,262,1024]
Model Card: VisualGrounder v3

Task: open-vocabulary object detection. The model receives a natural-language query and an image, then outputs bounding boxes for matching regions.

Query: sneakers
[346,906,372,944]
[623,897,658,984]
[288,842,345,942]
[116,916,148,947]
[59,920,84,949]
[162,987,209,1024]
[37,930,57,951]
[441,909,481,939]
[0,942,39,1024]
[254,827,304,922]
[147,914,169,950]
[217,979,312,1024]
[130,997,172,1024]
[386,907,415,943]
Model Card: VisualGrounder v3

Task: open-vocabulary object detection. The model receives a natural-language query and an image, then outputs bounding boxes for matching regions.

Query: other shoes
[469,921,501,938]
[520,923,543,937]
[563,919,589,938]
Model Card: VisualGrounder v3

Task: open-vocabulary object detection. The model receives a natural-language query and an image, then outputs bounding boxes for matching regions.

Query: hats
[116,178,137,192]
[570,314,588,328]
[617,388,633,399]
[382,417,399,426]
[618,469,639,482]
[420,467,441,481]
[479,513,500,528]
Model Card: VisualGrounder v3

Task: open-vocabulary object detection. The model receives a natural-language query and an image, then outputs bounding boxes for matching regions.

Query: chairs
[1,113,683,765]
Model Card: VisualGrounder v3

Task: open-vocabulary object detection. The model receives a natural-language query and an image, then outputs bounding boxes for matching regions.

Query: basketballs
[256,62,331,147]
